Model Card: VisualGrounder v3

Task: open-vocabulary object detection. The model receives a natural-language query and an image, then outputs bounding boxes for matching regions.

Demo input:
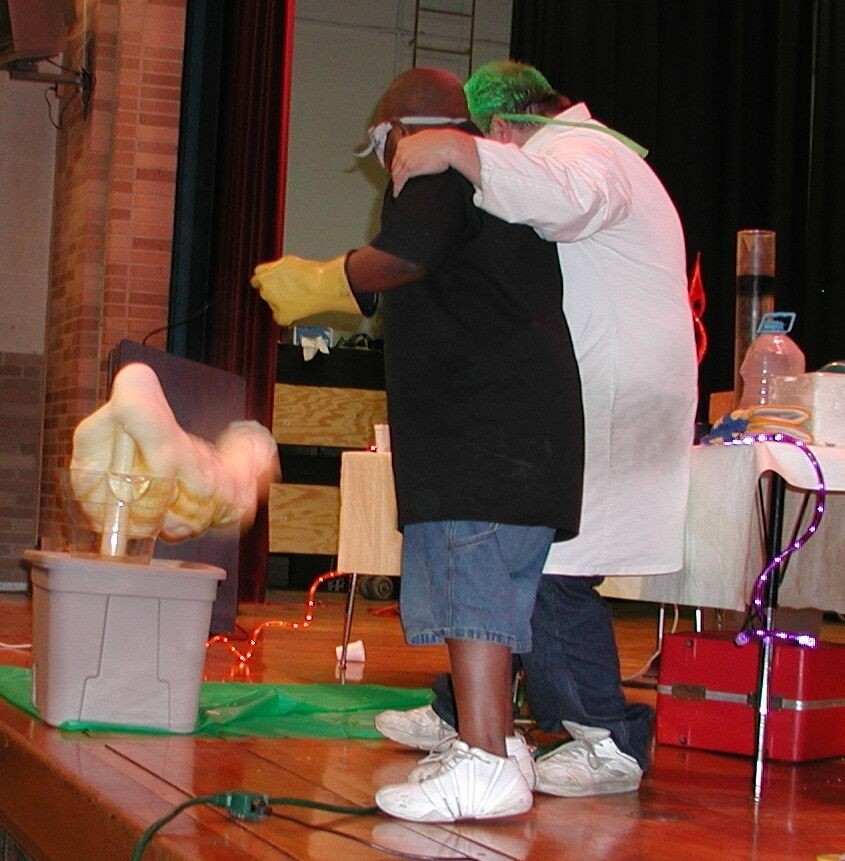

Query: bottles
[738,311,806,410]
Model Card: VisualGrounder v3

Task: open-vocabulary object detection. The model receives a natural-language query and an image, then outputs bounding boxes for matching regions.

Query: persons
[250,71,585,822]
[374,60,698,797]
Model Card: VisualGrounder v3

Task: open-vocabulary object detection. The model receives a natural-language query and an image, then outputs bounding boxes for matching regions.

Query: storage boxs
[802,372,845,444]
[656,630,845,763]
[20,549,227,734]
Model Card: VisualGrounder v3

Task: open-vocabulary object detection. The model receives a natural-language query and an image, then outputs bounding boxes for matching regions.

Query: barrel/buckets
[60,468,178,566]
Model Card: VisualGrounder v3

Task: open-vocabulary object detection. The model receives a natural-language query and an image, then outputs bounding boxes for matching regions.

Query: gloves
[701,406,814,445]
[251,249,379,326]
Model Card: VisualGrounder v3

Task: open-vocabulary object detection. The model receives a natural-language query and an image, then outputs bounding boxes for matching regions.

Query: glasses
[355,122,394,167]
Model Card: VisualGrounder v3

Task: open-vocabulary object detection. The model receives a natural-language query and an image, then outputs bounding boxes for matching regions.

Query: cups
[336,640,366,663]
[374,423,391,452]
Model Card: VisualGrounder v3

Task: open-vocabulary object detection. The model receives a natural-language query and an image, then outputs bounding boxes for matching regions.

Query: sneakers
[536,720,644,797]
[375,704,459,754]
[373,740,533,823]
[408,730,539,790]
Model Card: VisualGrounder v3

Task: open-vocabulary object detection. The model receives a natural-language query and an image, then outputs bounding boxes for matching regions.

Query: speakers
[0,0,64,70]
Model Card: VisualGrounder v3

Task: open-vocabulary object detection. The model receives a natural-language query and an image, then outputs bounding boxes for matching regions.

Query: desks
[338,443,845,803]
